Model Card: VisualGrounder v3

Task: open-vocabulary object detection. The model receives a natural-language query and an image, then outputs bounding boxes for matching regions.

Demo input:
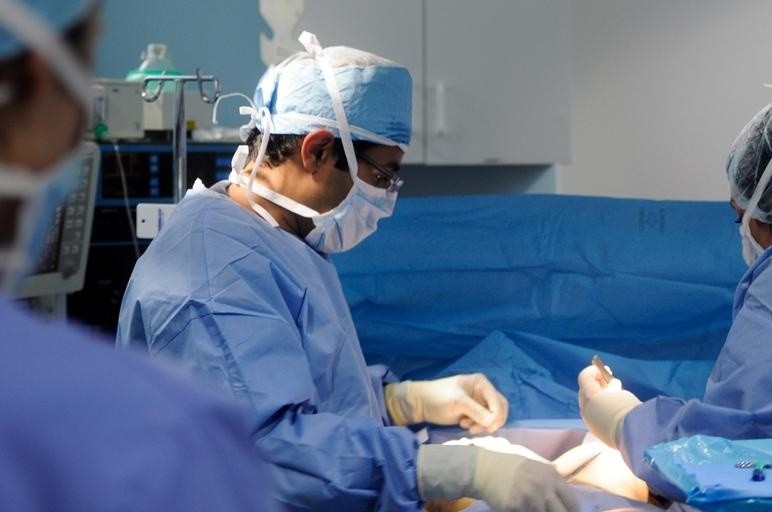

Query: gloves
[383,372,510,436]
[577,364,645,450]
[415,442,582,512]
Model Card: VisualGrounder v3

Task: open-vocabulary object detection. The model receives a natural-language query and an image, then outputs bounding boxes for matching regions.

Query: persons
[117,43,578,512]
[578,102,772,511]
[0,1,286,512]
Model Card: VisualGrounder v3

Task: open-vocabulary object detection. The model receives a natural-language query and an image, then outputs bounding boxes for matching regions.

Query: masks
[738,211,766,268]
[0,43,88,302]
[281,138,399,255]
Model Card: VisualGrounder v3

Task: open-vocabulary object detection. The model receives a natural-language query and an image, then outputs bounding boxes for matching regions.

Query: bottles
[139,44,170,70]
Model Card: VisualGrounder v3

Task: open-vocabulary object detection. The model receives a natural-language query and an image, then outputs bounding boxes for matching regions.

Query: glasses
[359,152,405,198]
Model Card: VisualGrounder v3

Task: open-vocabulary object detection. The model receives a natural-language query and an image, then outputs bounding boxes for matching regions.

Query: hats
[1,1,99,60]
[254,46,413,153]
[725,102,772,225]
